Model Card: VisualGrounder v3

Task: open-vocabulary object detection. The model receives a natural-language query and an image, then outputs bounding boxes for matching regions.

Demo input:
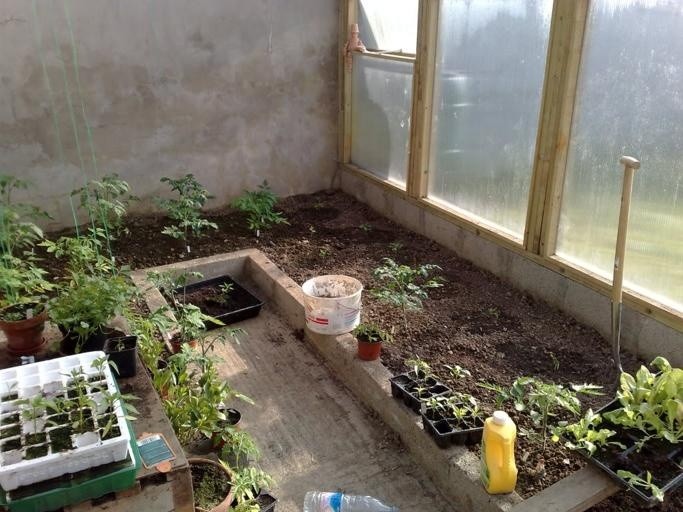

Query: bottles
[304,490,400,512]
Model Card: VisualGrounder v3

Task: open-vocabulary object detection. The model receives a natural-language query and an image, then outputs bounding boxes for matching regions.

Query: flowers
[0,178,48,320]
[350,318,392,343]
[147,267,198,338]
[39,228,138,343]
[155,343,255,442]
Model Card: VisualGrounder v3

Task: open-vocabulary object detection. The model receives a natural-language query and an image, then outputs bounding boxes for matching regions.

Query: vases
[250,487,278,512]
[218,407,243,448]
[169,337,197,354]
[0,302,47,352]
[56,321,103,353]
[103,336,138,378]
[185,457,237,512]
[357,336,381,360]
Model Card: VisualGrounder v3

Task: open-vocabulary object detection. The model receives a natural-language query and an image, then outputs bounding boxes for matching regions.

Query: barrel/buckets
[300,274,364,336]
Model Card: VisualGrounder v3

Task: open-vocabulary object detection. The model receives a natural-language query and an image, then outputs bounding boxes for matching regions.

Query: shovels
[603,154,640,385]
